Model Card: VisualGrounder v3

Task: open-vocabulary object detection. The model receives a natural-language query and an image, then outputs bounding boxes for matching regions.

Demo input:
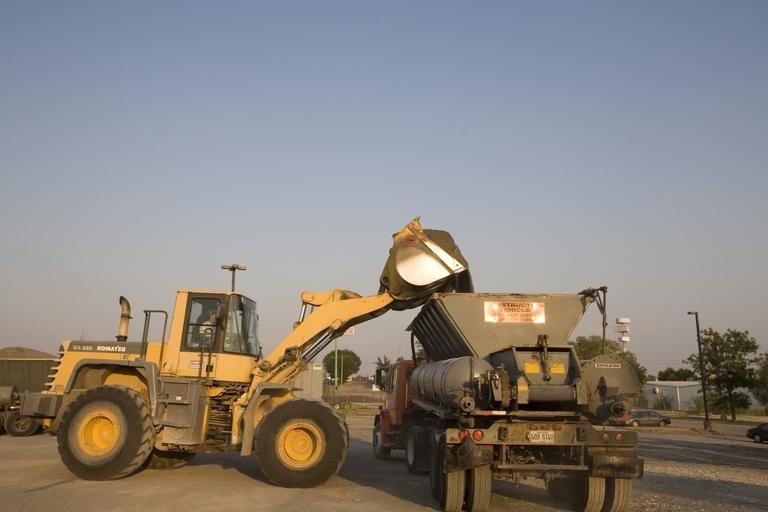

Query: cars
[625,406,671,428]
[747,421,768,443]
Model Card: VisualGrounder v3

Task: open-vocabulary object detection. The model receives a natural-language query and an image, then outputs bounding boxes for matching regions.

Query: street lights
[687,311,712,431]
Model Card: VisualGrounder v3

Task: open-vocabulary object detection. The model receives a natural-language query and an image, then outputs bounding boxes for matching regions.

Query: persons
[199,308,217,345]
